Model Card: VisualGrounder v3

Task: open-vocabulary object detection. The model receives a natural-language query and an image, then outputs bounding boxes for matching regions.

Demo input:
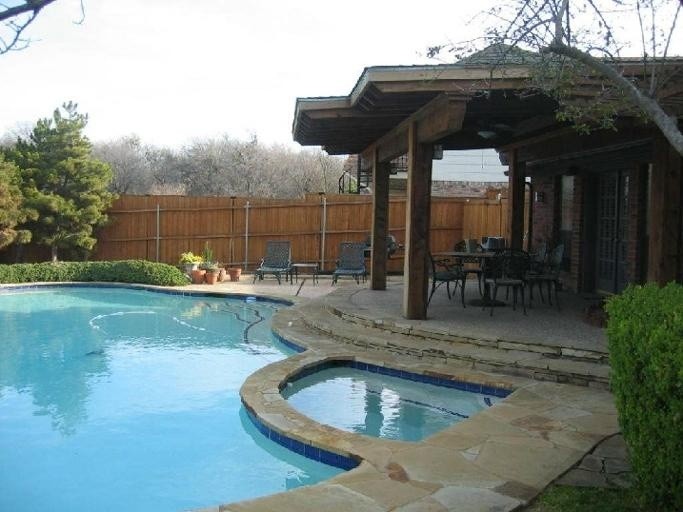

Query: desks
[290,263,318,287]
[435,250,537,308]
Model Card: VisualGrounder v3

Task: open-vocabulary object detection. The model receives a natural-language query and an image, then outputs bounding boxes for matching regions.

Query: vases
[191,266,241,287]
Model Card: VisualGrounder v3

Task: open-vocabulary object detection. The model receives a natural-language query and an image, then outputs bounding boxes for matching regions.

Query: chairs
[483,250,528,316]
[427,250,465,309]
[450,239,485,296]
[330,243,367,287]
[250,240,293,285]
[525,238,567,308]
